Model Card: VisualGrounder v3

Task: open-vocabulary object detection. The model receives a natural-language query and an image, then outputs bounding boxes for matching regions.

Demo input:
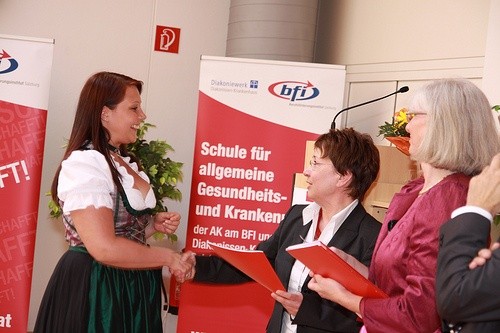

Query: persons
[307,80,500,333]
[433,152,500,333]
[34,72,195,333]
[172,128,384,333]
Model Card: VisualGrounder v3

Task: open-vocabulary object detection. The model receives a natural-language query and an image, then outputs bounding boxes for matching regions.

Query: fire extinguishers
[161,248,185,315]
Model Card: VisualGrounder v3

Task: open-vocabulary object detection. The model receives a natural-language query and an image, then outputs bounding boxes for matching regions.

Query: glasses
[310,159,330,167]
[405,111,427,123]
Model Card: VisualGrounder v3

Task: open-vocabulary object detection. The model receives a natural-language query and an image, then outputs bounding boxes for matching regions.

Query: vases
[386,136,417,179]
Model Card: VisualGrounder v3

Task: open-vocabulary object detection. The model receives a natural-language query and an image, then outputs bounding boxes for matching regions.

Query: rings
[186,273,189,278]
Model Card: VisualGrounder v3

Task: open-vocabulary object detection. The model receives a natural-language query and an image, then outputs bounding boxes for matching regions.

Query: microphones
[331,86,409,130]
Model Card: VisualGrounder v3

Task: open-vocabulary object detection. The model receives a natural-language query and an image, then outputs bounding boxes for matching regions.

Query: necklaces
[110,144,119,153]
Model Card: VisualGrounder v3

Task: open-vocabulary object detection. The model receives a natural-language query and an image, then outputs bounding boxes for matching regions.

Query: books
[284,241,390,299]
[208,242,286,293]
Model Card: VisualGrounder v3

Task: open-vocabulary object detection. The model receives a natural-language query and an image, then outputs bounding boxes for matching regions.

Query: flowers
[375,108,416,140]
